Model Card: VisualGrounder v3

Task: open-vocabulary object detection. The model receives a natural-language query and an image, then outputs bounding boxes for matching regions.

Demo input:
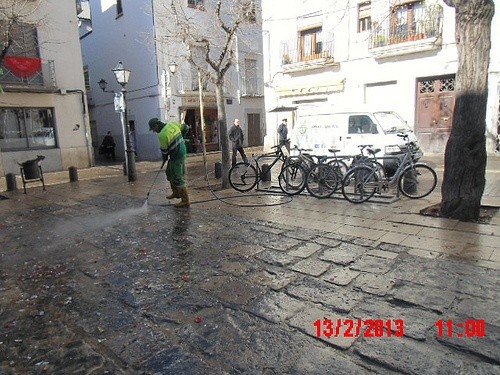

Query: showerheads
[144,191,150,201]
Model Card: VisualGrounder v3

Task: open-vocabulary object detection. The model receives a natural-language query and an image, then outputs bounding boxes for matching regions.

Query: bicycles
[228,139,439,204]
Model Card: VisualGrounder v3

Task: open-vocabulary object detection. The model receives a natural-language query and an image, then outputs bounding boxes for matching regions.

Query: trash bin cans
[20,160,46,194]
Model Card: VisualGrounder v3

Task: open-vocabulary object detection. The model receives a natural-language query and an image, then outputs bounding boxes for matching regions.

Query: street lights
[111,60,140,182]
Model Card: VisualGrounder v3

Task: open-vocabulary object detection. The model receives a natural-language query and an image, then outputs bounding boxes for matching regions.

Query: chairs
[19,160,46,194]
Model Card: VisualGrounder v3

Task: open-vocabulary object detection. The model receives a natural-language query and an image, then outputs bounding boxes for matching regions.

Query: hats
[148,118,160,130]
[282,119,287,121]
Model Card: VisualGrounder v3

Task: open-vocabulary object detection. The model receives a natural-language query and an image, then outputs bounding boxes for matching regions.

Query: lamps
[97,78,114,93]
[168,58,178,76]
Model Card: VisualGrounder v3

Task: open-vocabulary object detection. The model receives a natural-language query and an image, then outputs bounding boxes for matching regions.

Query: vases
[413,32,424,41]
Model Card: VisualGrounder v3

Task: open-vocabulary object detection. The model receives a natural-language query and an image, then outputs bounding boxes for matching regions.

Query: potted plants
[405,29,414,41]
[372,33,386,48]
[283,54,291,65]
[389,33,408,44]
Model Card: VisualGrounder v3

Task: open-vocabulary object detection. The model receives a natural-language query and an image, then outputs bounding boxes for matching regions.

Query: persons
[102,131,117,162]
[278,118,288,145]
[229,120,248,168]
[149,118,190,208]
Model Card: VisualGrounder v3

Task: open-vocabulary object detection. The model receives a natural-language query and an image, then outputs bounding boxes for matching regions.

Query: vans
[297,108,424,172]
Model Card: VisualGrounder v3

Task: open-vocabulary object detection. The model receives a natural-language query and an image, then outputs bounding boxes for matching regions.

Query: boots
[174,187,190,207]
[232,162,239,168]
[167,189,179,199]
